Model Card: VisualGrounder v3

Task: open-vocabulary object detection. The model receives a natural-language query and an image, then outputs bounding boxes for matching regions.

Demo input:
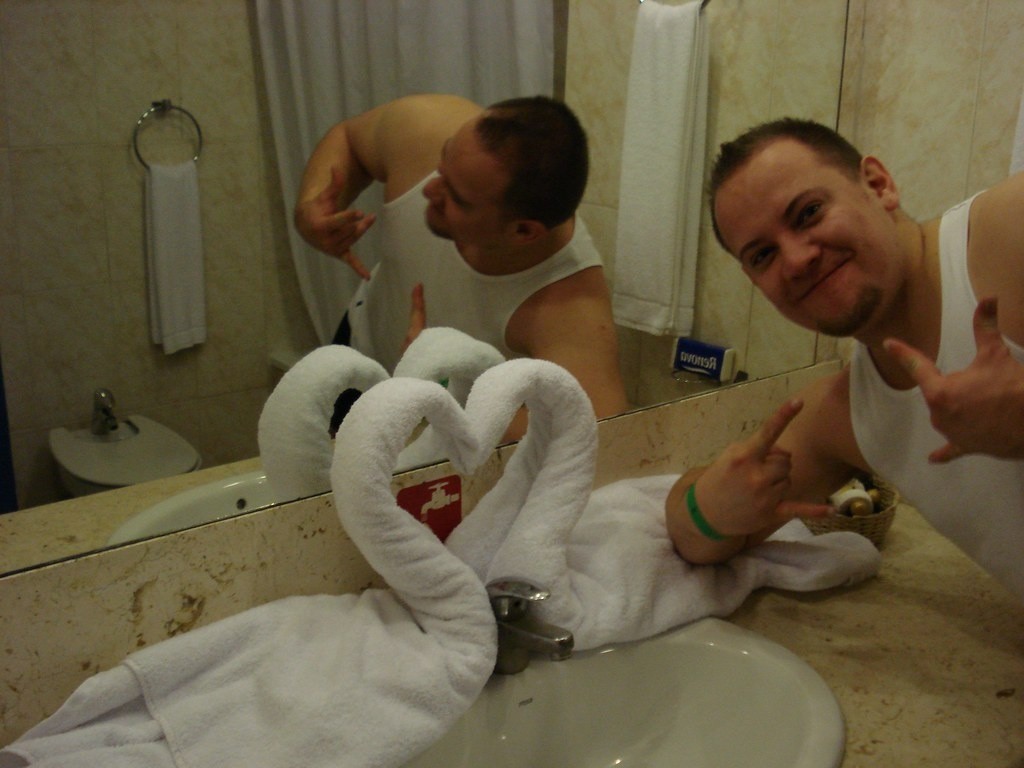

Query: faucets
[484,576,576,674]
[90,388,118,437]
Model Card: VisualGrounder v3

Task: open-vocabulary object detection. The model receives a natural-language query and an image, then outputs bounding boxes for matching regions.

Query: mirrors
[0,0,847,576]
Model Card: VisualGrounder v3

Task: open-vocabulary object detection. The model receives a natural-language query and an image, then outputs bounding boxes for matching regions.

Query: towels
[0,377,476,767]
[143,159,204,358]
[393,326,501,477]
[606,2,715,339]
[258,343,389,505]
[458,355,881,660]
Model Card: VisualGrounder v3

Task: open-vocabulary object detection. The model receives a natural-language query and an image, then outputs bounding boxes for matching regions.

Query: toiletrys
[833,477,871,515]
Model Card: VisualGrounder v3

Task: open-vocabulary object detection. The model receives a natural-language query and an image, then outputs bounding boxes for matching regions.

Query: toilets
[47,410,204,496]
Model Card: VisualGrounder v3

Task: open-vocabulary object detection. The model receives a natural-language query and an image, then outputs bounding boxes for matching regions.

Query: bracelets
[686,483,730,541]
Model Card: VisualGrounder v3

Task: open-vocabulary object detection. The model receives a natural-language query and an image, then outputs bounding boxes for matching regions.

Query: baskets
[803,475,898,547]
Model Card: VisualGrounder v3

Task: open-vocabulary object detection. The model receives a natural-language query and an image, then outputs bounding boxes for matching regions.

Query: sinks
[117,473,269,550]
[413,614,855,766]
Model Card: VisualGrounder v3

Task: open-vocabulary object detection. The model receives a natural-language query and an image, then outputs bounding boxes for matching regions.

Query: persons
[296,94,630,447]
[666,116,1024,613]
